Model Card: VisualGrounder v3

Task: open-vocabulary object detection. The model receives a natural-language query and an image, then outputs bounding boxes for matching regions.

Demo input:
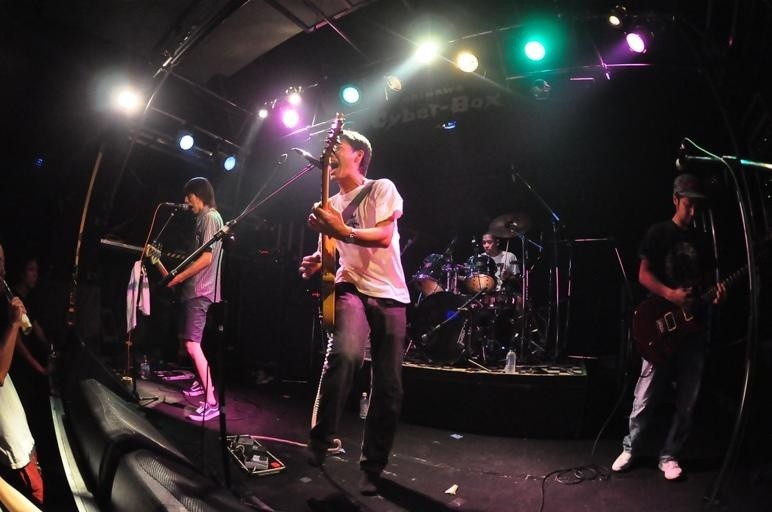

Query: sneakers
[189,402,220,422]
[358,471,379,494]
[659,456,682,480]
[611,450,634,472]
[182,381,215,396]
[308,448,325,466]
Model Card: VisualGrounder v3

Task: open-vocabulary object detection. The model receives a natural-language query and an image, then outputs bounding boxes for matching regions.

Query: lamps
[212,150,236,171]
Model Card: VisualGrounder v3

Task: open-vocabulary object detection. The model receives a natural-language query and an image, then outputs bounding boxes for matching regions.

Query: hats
[673,175,707,199]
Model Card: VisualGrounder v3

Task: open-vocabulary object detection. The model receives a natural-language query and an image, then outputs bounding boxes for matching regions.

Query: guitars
[145,241,183,322]
[317,113,344,324]
[632,259,763,365]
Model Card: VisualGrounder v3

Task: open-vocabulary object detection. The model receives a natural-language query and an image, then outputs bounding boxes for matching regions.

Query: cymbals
[488,213,530,238]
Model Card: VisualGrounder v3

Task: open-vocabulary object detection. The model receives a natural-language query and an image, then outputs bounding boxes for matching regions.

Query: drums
[465,255,498,292]
[410,292,485,364]
[413,254,455,296]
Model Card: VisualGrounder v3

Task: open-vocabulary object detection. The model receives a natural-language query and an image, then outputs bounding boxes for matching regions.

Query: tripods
[503,243,548,362]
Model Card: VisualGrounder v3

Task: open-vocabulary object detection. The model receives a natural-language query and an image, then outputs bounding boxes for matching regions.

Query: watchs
[343,227,355,244]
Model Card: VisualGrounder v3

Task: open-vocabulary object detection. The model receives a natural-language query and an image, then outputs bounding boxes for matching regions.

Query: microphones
[675,143,693,173]
[162,202,188,211]
[421,325,441,343]
[293,146,332,173]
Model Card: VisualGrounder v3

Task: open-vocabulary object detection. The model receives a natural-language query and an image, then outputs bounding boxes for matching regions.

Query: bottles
[3,280,33,335]
[358,392,370,422]
[505,346,517,372]
[139,354,150,381]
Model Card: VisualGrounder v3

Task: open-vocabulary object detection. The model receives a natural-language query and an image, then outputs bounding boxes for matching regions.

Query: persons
[444,233,538,368]
[609,175,729,481]
[297,129,411,494]
[6,254,55,434]
[0,295,50,511]
[166,177,225,420]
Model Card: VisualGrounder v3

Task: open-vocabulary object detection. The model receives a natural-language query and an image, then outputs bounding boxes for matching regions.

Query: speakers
[62,378,190,494]
[110,447,260,512]
[64,355,131,397]
